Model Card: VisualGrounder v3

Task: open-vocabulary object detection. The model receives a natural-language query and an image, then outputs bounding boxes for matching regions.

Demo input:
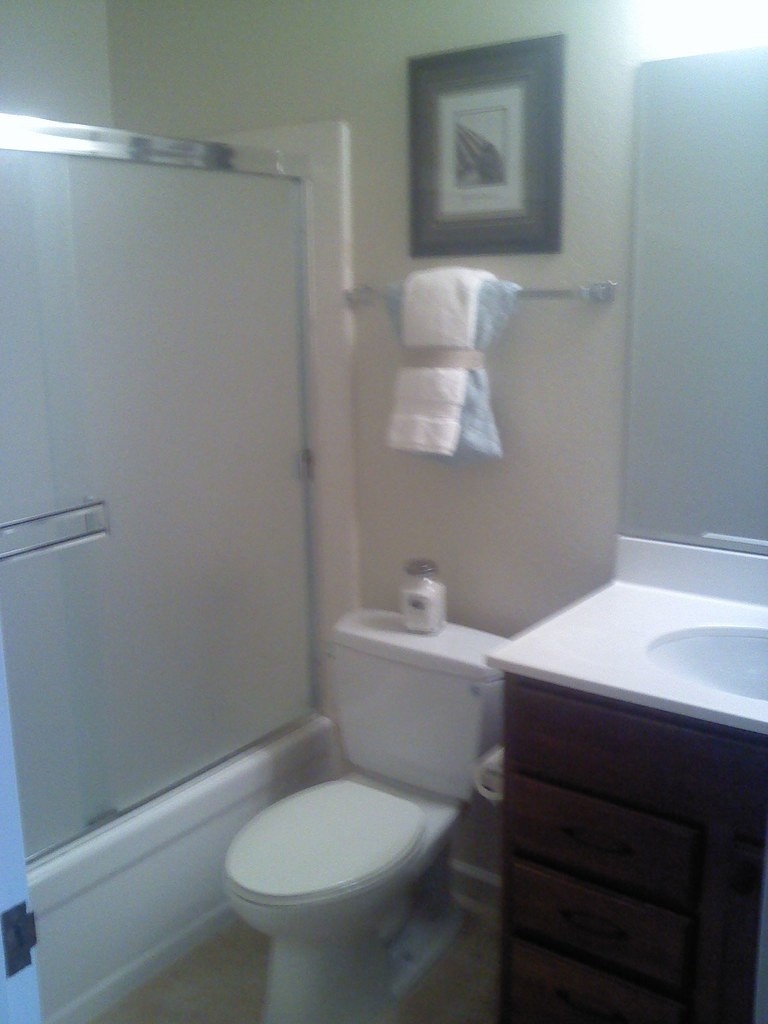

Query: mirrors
[621,47,767,559]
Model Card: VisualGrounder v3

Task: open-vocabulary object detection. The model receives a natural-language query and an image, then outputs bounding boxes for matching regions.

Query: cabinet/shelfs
[504,670,767,1024]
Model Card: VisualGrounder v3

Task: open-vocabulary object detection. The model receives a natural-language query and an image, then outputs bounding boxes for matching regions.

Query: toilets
[226,611,510,1024]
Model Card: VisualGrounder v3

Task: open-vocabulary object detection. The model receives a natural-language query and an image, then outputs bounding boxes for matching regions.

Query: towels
[387,268,514,463]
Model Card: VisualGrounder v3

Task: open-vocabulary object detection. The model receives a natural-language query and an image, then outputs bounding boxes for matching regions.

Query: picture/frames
[406,31,564,260]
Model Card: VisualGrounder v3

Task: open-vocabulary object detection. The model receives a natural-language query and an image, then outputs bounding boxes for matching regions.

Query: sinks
[650,627,766,704]
